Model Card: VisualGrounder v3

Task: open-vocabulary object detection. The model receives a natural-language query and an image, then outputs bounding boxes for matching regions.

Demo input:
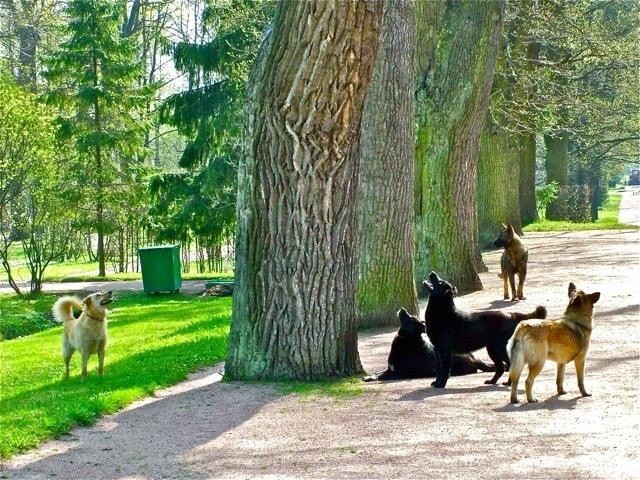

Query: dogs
[491,220,529,303]
[421,270,547,388]
[507,281,601,404]
[363,307,508,382]
[52,290,114,383]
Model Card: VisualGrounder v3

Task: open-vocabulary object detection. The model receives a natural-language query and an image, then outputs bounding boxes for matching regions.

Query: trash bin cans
[609,181,617,189]
[138,245,181,294]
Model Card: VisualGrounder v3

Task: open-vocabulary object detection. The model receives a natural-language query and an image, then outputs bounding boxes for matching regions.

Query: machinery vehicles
[627,169,640,186]
